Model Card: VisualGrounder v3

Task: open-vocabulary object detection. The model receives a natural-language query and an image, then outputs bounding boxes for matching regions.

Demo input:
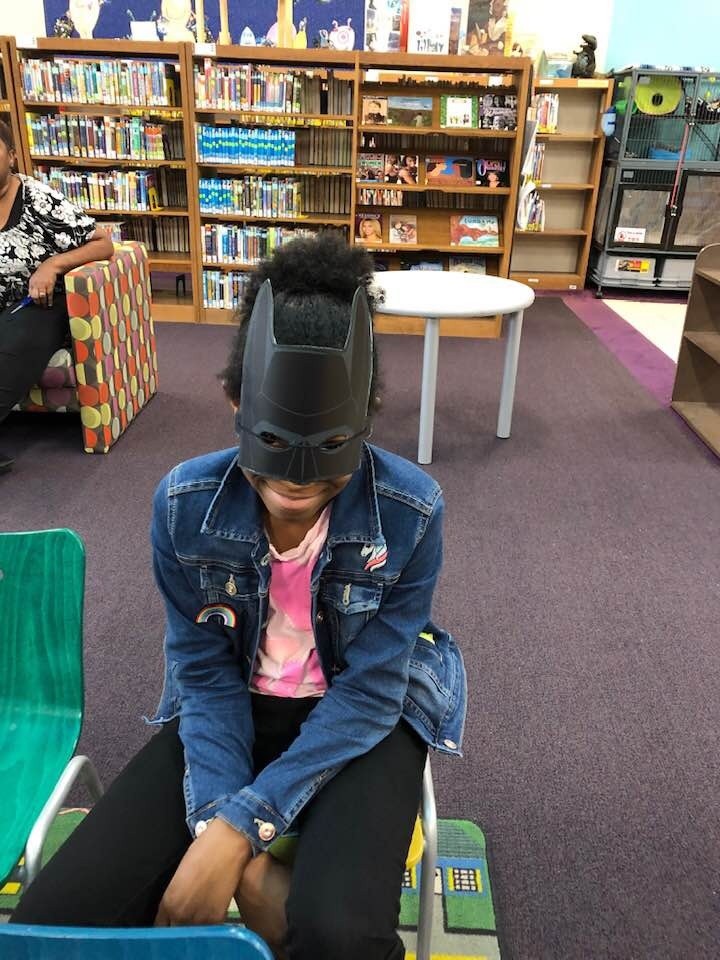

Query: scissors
[10,298,32,315]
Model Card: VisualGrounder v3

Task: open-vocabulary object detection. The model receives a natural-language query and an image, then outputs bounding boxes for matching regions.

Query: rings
[40,292,46,296]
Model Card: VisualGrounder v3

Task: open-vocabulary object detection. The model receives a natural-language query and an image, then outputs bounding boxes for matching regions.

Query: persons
[389,221,417,243]
[487,171,503,187]
[385,154,401,183]
[358,219,382,244]
[13,229,470,960]
[364,101,387,126]
[399,155,417,184]
[0,117,114,474]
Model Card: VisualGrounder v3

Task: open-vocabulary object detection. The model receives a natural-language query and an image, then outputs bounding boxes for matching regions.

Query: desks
[365,267,537,465]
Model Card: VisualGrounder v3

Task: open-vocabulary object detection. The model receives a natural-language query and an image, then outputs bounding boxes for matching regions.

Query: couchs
[9,239,163,456]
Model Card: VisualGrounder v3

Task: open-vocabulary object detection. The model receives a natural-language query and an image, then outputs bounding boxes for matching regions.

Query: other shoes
[0,453,16,467]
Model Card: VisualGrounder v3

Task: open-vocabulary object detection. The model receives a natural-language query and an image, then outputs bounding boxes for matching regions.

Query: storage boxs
[590,250,695,290]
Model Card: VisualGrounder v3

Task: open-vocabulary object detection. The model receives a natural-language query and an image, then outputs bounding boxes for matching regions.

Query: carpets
[0,303,720,960]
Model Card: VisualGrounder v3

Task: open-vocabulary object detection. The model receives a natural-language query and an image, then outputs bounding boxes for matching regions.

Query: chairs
[0,526,106,901]
[268,748,467,960]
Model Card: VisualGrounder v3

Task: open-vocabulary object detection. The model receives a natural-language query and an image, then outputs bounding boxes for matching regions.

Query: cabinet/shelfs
[511,69,614,296]
[583,66,720,300]
[668,242,720,458]
[0,32,533,324]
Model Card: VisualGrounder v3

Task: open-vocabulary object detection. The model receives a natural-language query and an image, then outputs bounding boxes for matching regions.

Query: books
[33,165,189,213]
[197,122,352,309]
[23,56,189,105]
[194,61,354,116]
[26,113,184,161]
[119,217,187,253]
[357,67,558,274]
